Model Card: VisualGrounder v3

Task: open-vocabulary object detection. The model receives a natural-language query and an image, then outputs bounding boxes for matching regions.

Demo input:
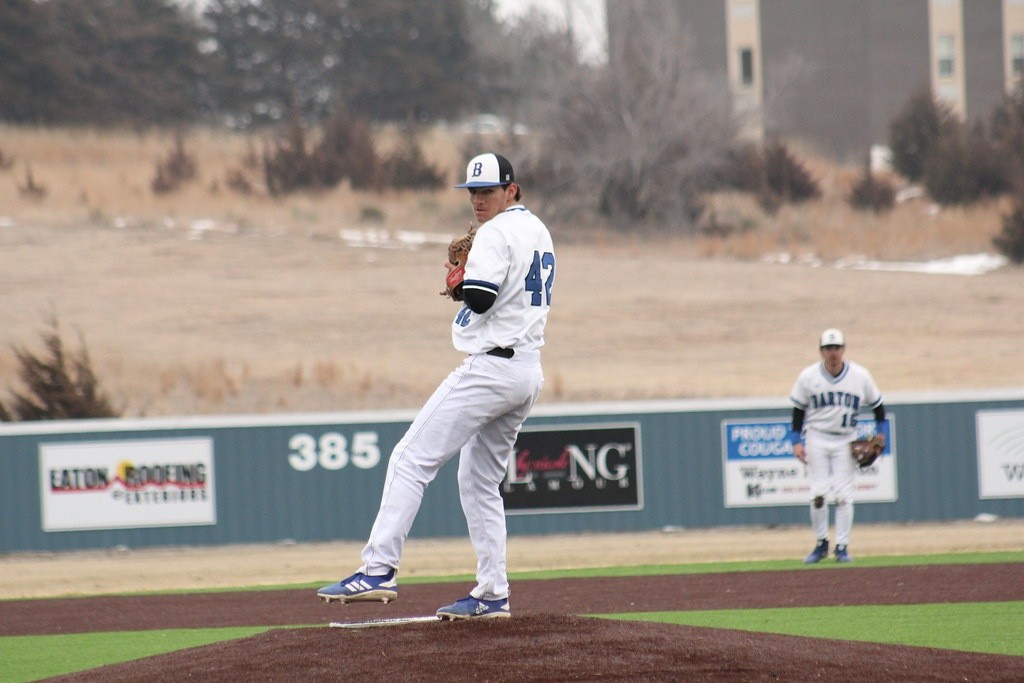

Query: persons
[789,328,890,566]
[314,152,557,623]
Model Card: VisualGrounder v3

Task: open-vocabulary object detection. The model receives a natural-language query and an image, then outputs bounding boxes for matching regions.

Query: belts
[469,347,515,359]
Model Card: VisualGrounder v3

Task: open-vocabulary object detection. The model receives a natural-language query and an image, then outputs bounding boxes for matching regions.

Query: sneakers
[315,568,398,606]
[435,593,512,620]
[805,538,831,565]
[833,544,852,563]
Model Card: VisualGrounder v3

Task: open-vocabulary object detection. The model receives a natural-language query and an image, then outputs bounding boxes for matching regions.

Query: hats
[819,328,844,348]
[454,152,514,188]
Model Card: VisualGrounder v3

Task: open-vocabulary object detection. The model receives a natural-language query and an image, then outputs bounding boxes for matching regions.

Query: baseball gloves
[440,221,478,302]
[851,435,886,469]
